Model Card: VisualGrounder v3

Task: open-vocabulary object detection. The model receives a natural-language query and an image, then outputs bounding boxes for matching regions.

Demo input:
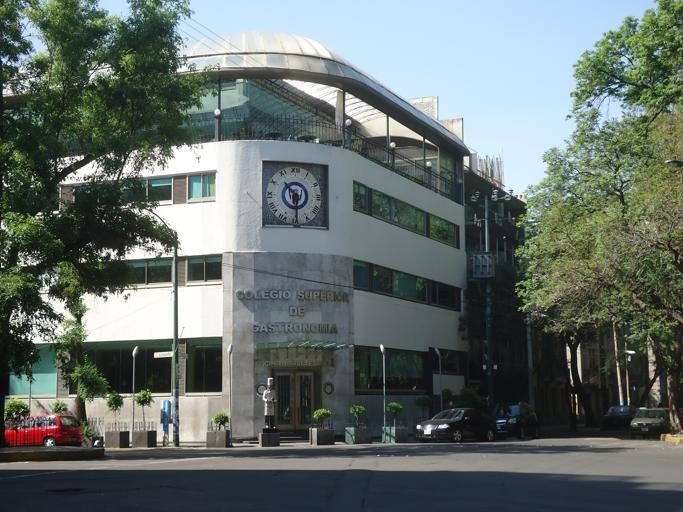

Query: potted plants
[382,402,408,444]
[206,411,231,448]
[309,408,336,445]
[105,390,129,448]
[344,403,372,445]
[132,389,157,448]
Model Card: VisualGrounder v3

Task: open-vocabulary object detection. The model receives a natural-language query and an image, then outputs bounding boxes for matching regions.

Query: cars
[416,402,538,443]
[603,406,670,439]
[5,414,82,446]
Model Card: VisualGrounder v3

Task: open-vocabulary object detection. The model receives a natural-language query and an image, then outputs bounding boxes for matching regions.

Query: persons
[261,384,278,428]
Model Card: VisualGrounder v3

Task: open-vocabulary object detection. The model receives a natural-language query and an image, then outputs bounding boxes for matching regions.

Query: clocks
[262,159,330,231]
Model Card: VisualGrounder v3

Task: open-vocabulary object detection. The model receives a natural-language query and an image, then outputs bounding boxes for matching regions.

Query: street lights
[430,344,443,410]
[131,346,138,430]
[380,343,386,443]
[227,344,233,446]
